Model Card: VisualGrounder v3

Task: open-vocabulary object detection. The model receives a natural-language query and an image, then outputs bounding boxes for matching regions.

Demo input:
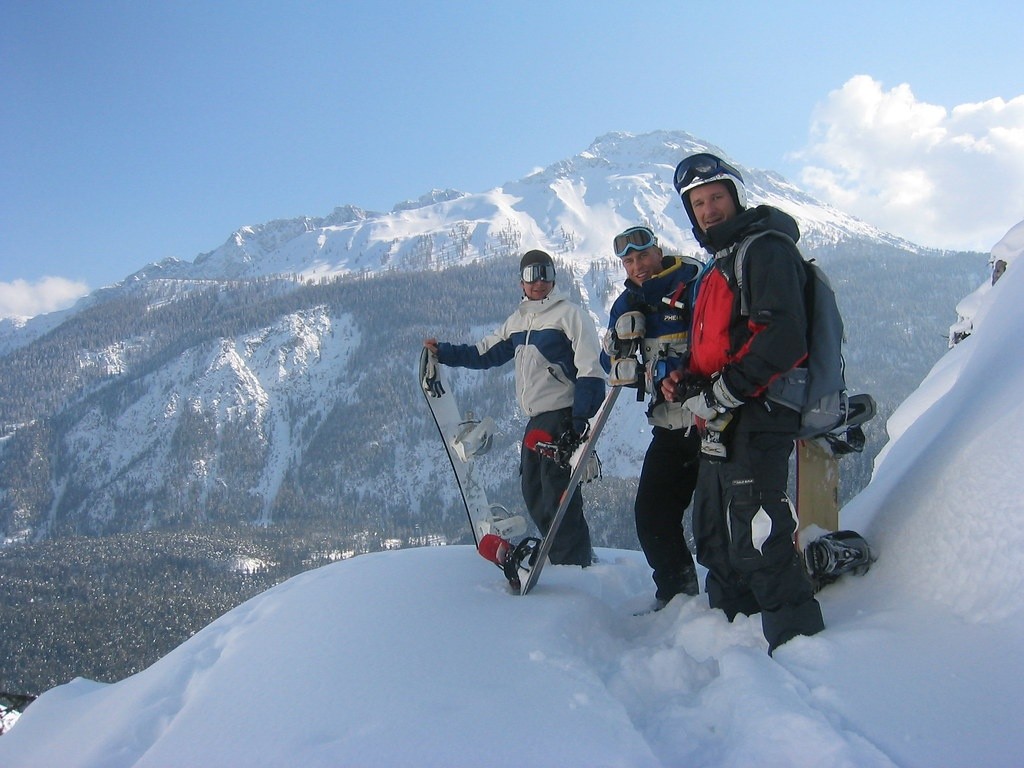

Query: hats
[520,249,553,271]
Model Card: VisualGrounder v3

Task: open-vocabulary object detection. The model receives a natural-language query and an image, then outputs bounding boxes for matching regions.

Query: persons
[599,227,734,616]
[423,250,607,567]
[659,153,825,655]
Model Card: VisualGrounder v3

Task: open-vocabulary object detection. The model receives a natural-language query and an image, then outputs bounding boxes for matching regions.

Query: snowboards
[419,346,526,550]
[499,341,637,596]
[795,393,877,596]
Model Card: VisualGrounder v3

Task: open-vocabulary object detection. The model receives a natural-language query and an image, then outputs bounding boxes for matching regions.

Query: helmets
[675,153,749,210]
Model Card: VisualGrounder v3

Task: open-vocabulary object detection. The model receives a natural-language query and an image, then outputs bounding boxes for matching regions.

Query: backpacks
[731,228,875,456]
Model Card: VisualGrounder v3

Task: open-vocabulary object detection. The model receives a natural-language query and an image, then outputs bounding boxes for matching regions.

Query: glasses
[675,156,720,187]
[614,230,655,256]
[521,264,558,283]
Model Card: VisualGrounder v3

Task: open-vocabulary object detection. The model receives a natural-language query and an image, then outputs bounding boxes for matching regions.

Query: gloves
[682,371,745,421]
[422,350,446,399]
[570,439,598,484]
[706,411,735,443]
